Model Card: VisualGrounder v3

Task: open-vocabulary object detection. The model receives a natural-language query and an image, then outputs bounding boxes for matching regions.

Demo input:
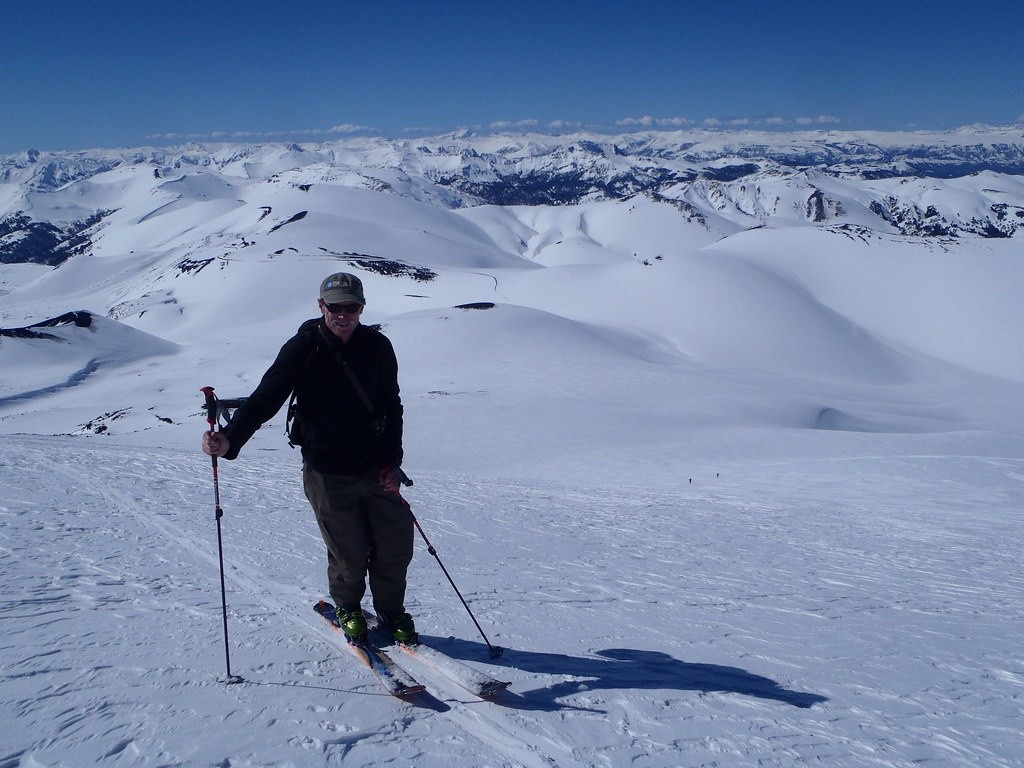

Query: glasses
[322,302,361,314]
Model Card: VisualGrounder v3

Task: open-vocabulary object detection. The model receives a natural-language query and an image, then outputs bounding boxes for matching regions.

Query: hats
[319,272,366,306]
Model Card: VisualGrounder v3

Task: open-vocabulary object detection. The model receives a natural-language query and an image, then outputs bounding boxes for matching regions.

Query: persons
[203,272,417,645]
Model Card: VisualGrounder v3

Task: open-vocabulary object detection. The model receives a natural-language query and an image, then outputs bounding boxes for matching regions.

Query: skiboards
[312,599,512,699]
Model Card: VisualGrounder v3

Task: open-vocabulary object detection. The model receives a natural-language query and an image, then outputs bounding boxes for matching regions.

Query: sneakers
[335,606,368,643]
[377,613,419,648]
